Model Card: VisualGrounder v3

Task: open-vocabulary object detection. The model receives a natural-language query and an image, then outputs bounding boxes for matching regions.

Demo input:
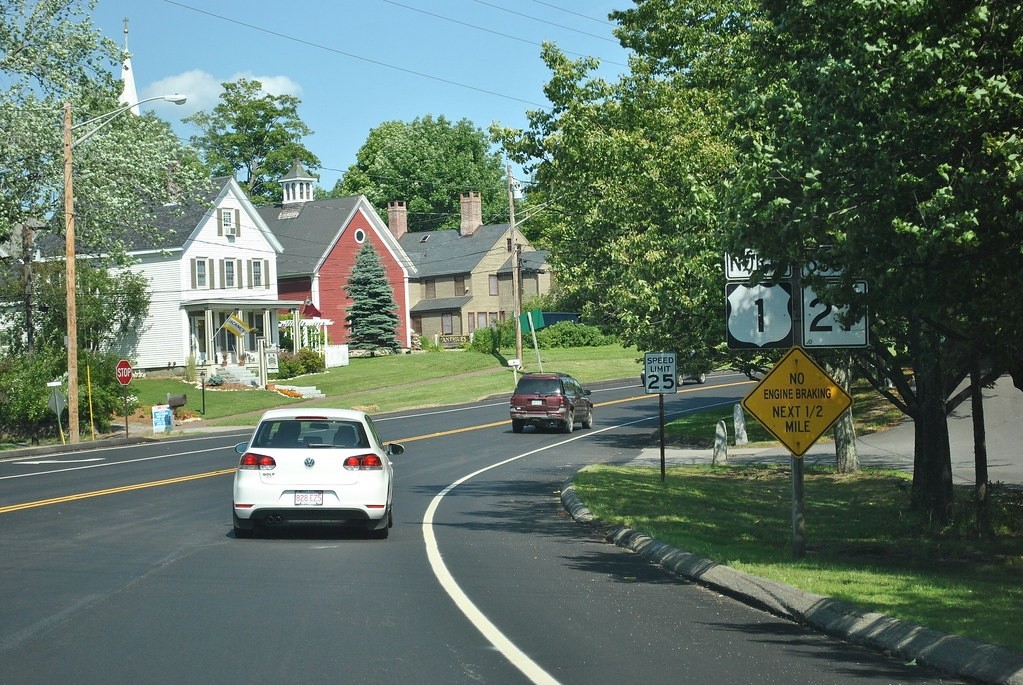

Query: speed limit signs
[644,352,678,394]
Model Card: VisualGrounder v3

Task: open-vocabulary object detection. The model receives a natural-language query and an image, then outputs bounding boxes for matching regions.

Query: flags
[222,315,251,339]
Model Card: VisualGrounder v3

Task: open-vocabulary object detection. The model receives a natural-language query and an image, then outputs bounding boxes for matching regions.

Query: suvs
[510,372,594,434]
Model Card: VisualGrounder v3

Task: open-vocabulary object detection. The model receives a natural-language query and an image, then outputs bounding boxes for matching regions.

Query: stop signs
[115,360,133,386]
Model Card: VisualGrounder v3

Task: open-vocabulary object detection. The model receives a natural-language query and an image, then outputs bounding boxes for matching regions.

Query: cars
[639,370,708,387]
[232,407,405,539]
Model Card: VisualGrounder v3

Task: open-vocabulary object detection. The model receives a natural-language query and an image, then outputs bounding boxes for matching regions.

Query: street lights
[64,93,189,444]
